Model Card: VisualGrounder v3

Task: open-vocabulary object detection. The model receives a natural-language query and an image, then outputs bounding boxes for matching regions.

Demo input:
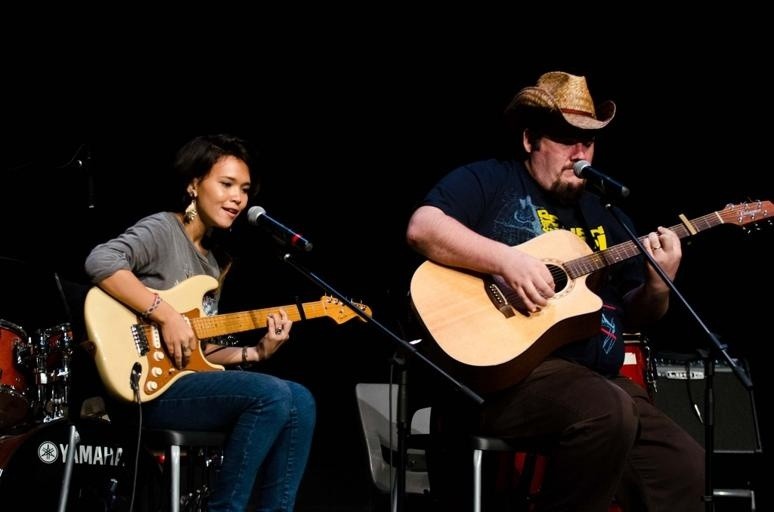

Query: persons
[81,131,319,512]
[401,69,711,511]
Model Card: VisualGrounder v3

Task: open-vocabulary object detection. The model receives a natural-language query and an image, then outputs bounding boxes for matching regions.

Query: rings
[182,345,190,352]
[652,245,662,251]
[275,327,283,334]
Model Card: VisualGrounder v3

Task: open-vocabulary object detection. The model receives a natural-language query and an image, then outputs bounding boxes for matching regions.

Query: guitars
[84,275,372,404]
[411,200,773,388]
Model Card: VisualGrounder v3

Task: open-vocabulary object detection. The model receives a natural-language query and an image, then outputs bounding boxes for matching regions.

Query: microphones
[572,159,630,198]
[246,205,314,253]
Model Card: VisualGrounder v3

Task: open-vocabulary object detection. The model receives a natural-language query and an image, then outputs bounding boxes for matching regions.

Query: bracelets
[242,343,250,366]
[140,292,162,321]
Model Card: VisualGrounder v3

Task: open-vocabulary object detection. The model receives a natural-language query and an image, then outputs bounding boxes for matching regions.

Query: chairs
[54,274,227,512]
[398,358,550,511]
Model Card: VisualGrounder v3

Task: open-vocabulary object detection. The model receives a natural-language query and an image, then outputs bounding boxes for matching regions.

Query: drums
[511,330,657,498]
[2,317,32,423]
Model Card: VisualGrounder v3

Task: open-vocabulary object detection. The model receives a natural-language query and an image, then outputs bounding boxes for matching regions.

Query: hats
[508,71,618,130]
